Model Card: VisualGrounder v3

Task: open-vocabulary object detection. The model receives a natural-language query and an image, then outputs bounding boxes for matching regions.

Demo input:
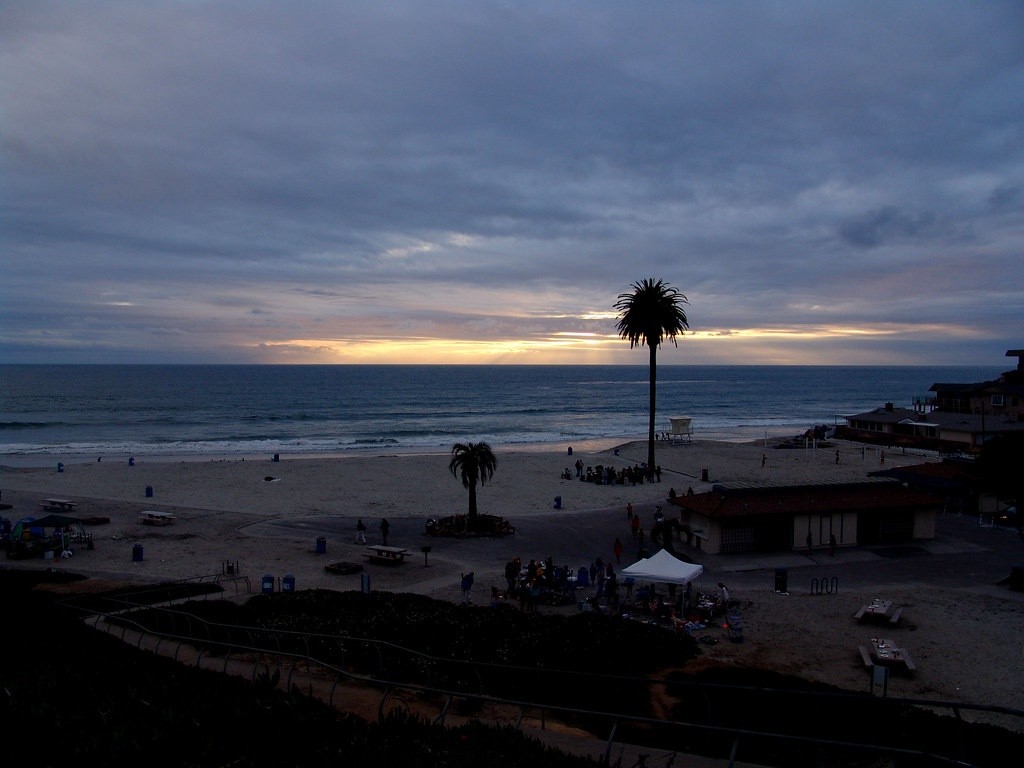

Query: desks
[865,600,895,615]
[367,545,407,558]
[871,638,908,661]
[858,646,874,666]
[899,648,917,672]
[42,498,72,508]
[141,511,173,519]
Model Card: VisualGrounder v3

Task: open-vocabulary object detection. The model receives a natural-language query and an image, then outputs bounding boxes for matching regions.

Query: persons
[626,503,633,520]
[380,518,390,546]
[614,538,624,565]
[460,571,474,604]
[669,488,676,498]
[829,535,837,556]
[575,459,661,486]
[762,454,767,468]
[631,514,645,546]
[53,522,76,536]
[880,450,885,465]
[687,487,694,497]
[653,505,664,520]
[354,519,366,545]
[806,532,813,556]
[504,556,730,622]
[655,432,665,441]
[835,450,840,464]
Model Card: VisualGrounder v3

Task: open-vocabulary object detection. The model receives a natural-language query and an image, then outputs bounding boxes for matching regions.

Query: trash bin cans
[701,468,710,481]
[568,446,572,455]
[132,542,144,561]
[262,574,275,594]
[283,574,295,591]
[58,462,64,473]
[145,485,154,497]
[129,457,135,466]
[316,536,327,553]
[554,495,561,509]
[426,518,436,536]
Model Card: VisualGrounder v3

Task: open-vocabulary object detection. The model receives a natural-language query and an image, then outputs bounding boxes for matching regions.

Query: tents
[620,548,702,618]
[11,518,44,541]
[21,515,81,551]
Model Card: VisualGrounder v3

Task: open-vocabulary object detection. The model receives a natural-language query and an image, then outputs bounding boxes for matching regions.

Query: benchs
[889,608,904,624]
[385,552,413,560]
[139,517,162,526]
[167,516,176,523]
[39,503,61,511]
[65,503,78,509]
[362,554,394,566]
[854,604,868,620]
